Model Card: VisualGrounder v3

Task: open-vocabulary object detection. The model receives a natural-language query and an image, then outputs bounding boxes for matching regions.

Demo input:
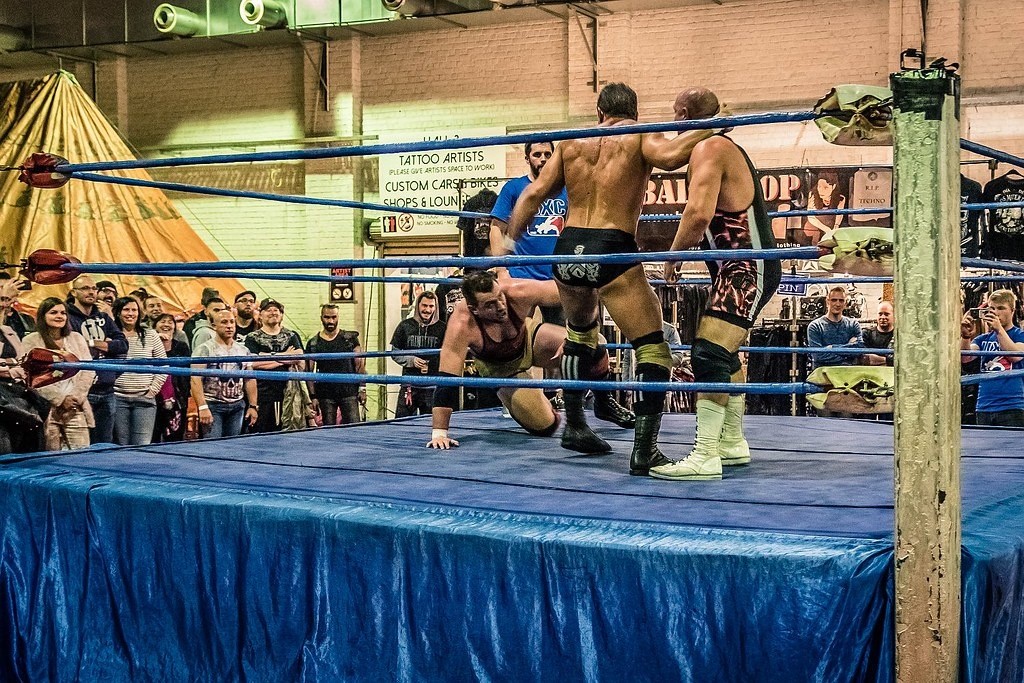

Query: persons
[390,292,446,421]
[425,272,634,449]
[649,87,782,481]
[961,289,1024,432]
[0,273,315,454]
[807,288,862,372]
[622,320,683,413]
[862,302,894,367]
[500,82,732,475]
[489,141,569,406]
[803,169,845,249]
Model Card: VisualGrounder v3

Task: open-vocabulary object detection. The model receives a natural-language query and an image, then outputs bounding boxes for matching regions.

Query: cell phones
[971,307,995,321]
[14,280,32,290]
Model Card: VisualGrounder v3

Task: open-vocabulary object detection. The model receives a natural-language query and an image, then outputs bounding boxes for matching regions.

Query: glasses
[99,289,118,296]
[238,298,255,303]
[72,286,100,293]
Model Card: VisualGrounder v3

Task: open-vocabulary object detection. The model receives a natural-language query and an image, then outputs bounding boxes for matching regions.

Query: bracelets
[309,393,316,399]
[357,386,366,394]
[961,333,970,340]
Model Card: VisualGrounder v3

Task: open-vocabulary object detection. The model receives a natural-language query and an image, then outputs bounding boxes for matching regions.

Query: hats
[95,280,117,291]
[234,291,256,303]
[202,287,219,296]
[259,297,281,312]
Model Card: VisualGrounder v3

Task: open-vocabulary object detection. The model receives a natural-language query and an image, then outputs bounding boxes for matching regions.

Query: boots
[559,392,613,453]
[716,391,752,465]
[628,402,678,476]
[648,398,727,481]
[588,372,636,429]
